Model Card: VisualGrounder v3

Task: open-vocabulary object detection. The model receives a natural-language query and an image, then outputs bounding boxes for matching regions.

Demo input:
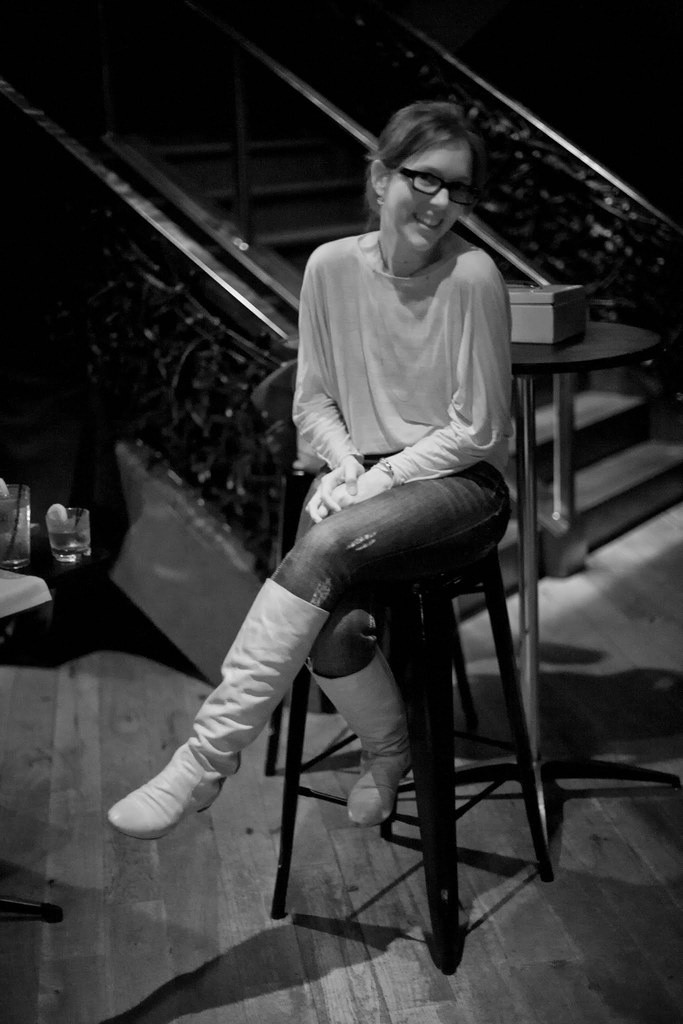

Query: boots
[304,642,415,826]
[107,577,330,841]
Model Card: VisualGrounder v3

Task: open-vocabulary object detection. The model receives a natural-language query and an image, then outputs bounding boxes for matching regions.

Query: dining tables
[0,539,104,927]
[501,324,664,522]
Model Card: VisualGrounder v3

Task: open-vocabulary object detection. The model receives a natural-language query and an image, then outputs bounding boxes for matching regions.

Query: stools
[266,458,552,973]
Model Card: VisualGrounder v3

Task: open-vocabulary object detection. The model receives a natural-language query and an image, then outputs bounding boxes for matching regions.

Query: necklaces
[376,236,445,276]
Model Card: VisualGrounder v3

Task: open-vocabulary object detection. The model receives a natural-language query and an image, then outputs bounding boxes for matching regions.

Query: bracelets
[378,458,395,479]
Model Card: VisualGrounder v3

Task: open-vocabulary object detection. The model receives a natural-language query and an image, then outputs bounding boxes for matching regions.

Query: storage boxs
[511,285,588,344]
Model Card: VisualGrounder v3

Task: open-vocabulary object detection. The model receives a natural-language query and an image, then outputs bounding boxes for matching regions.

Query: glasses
[387,164,482,205]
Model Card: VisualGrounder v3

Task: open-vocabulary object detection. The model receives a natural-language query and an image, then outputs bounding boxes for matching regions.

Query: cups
[46,507,90,562]
[0,484,31,570]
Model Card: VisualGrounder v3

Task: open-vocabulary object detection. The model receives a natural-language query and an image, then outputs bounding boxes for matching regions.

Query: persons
[106,101,513,840]
